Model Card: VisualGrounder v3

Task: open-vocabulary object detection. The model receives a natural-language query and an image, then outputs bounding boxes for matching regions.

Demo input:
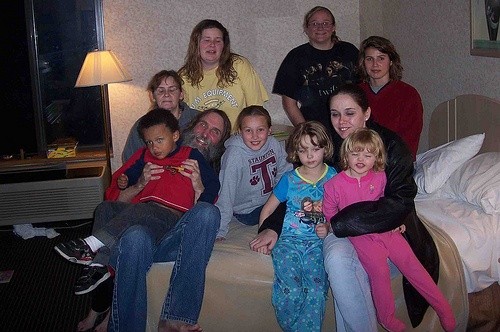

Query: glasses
[309,22,333,27]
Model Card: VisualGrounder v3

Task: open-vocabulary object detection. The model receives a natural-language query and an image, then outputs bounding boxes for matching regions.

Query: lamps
[75,50,134,187]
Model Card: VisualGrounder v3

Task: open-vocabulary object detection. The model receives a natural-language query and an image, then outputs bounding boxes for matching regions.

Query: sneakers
[75,264,111,295]
[54,239,93,266]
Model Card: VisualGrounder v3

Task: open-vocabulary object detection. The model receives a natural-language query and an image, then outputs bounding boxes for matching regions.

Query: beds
[145,95,500,332]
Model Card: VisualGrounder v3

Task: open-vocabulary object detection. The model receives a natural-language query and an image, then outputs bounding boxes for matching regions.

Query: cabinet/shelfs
[0,159,111,227]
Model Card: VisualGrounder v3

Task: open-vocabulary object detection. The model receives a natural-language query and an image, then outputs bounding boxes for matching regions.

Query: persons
[53,37,458,332]
[177,19,269,137]
[272,6,360,135]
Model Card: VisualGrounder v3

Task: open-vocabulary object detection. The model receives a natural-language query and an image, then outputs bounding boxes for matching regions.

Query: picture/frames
[469,0,500,59]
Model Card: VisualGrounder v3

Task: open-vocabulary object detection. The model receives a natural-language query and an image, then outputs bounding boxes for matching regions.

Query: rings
[192,169,195,172]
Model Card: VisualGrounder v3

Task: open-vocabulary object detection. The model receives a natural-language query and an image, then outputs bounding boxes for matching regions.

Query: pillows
[435,151,500,217]
[411,132,486,194]
